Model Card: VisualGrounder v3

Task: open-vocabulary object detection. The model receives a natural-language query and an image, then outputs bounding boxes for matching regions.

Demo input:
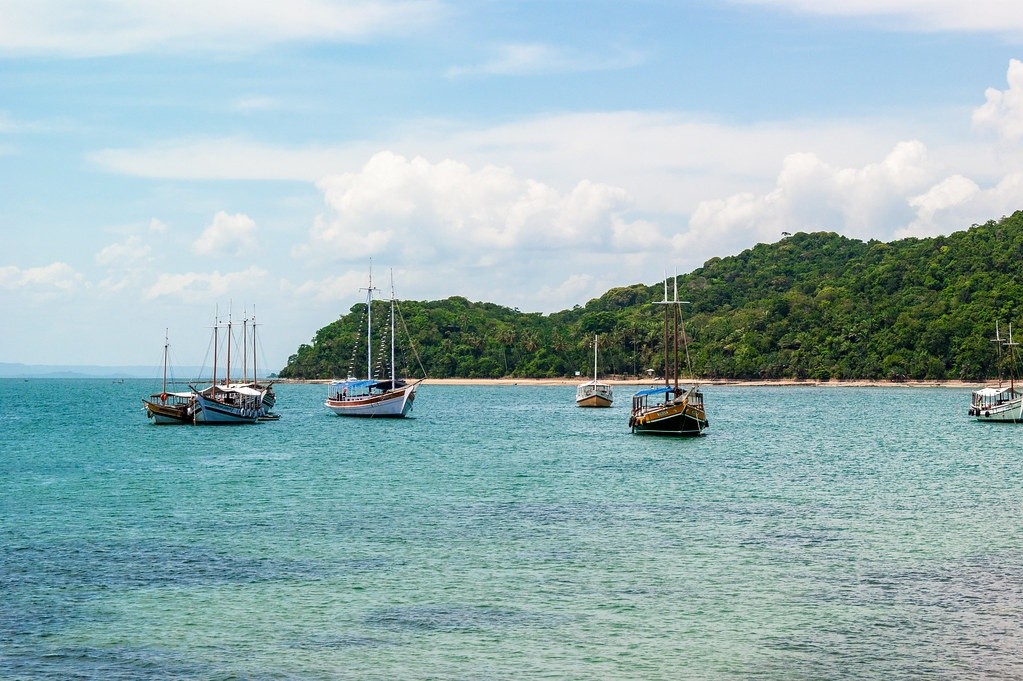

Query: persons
[336,392,342,401]
[343,386,347,397]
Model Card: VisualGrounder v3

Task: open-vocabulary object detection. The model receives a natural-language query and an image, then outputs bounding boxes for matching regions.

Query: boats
[257,412,281,420]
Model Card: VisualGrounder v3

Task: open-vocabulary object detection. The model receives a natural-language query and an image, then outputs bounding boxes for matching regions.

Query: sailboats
[188,298,276,424]
[969,318,1023,422]
[628,260,709,435]
[140,327,193,426]
[575,332,615,408]
[322,256,430,418]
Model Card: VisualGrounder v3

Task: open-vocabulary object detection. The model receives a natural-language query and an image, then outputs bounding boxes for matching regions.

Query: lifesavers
[240,408,267,418]
[628,416,634,428]
